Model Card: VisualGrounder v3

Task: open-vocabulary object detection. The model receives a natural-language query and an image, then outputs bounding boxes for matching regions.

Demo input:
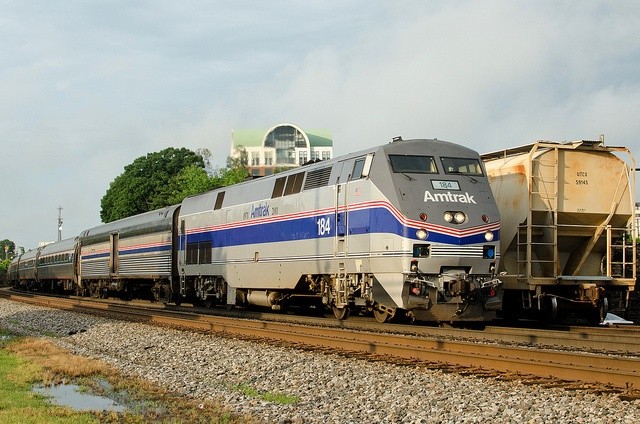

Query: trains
[7,136,502,322]
[479,133,636,322]
